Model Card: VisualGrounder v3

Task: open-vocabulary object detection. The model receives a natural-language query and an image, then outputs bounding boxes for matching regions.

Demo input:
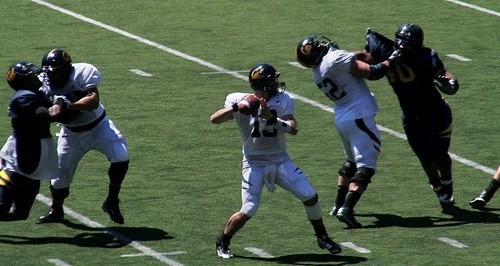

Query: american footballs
[237,95,260,116]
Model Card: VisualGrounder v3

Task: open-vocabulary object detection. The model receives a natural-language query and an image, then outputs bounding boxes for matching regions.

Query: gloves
[434,76,459,96]
[389,50,404,68]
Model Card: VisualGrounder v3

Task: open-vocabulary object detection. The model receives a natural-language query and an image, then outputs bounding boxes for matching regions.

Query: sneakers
[215,240,233,258]
[330,203,362,228]
[102,199,124,225]
[35,209,64,224]
[317,235,342,254]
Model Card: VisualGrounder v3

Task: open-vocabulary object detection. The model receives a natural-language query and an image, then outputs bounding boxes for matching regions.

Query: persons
[0,62,96,222]
[365,24,457,211]
[295,35,404,228]
[211,64,342,258]
[469,165,500,208]
[35,49,130,225]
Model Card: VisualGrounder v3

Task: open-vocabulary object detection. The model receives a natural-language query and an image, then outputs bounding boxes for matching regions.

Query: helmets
[41,49,71,91]
[297,36,328,69]
[249,63,280,89]
[7,62,43,91]
[394,22,424,57]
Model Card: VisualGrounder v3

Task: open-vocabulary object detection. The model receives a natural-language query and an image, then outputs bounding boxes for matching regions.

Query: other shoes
[440,196,454,203]
[470,197,486,208]
